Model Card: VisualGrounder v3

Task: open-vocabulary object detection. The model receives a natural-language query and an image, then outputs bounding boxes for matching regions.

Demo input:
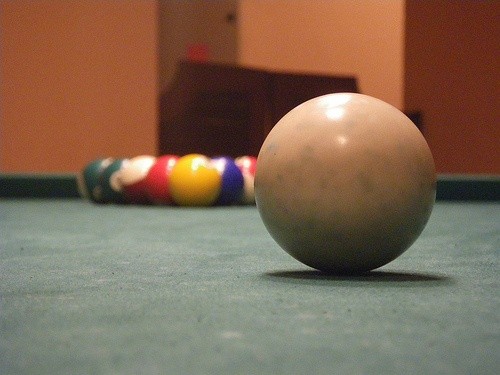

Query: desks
[0,170,500,375]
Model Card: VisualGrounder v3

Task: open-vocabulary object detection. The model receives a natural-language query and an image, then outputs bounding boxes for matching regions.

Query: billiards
[76,153,257,205]
[255,92,436,274]
